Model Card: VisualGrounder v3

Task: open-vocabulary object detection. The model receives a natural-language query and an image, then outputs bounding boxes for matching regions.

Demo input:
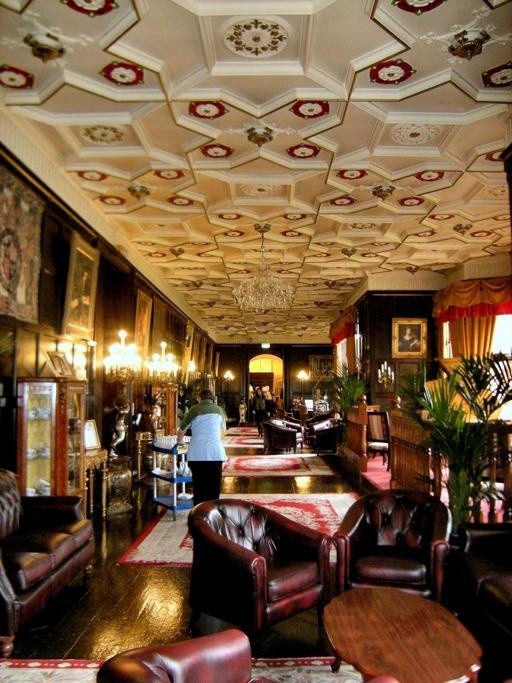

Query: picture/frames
[61,230,101,341]
[134,287,153,358]
[83,419,102,449]
[42,350,74,377]
[1,164,45,326]
[308,354,336,382]
[395,358,423,394]
[392,317,427,358]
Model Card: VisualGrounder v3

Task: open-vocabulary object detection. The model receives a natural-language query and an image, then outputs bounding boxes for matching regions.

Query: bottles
[176,461,192,477]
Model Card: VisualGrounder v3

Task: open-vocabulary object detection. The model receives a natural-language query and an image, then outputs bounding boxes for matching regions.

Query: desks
[322,587,483,683]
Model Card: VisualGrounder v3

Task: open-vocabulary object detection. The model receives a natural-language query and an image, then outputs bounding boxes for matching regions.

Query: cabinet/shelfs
[165,382,187,435]
[16,375,88,519]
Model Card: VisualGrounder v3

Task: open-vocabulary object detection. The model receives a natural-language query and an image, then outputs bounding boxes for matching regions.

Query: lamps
[103,329,143,386]
[146,341,186,388]
[231,222,296,312]
[377,360,394,388]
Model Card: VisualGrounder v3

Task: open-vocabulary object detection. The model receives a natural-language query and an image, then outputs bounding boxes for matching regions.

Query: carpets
[224,426,265,434]
[114,492,397,567]
[221,453,340,476]
[220,435,265,448]
[1,655,363,683]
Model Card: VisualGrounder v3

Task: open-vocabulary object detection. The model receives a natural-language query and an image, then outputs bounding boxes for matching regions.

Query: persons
[176,389,227,508]
[399,325,418,352]
[238,396,248,427]
[103,394,132,458]
[139,303,147,338]
[70,264,91,326]
[252,388,268,438]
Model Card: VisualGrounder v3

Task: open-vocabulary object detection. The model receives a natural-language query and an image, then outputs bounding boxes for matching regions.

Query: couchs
[0,468,96,657]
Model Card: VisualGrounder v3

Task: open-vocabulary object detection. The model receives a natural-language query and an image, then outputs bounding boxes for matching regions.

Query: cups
[155,434,192,448]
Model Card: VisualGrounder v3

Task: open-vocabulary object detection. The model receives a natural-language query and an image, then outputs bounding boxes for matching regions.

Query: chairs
[332,488,453,603]
[263,409,346,453]
[96,628,401,683]
[366,410,392,470]
[188,498,332,643]
[453,522,511,635]
